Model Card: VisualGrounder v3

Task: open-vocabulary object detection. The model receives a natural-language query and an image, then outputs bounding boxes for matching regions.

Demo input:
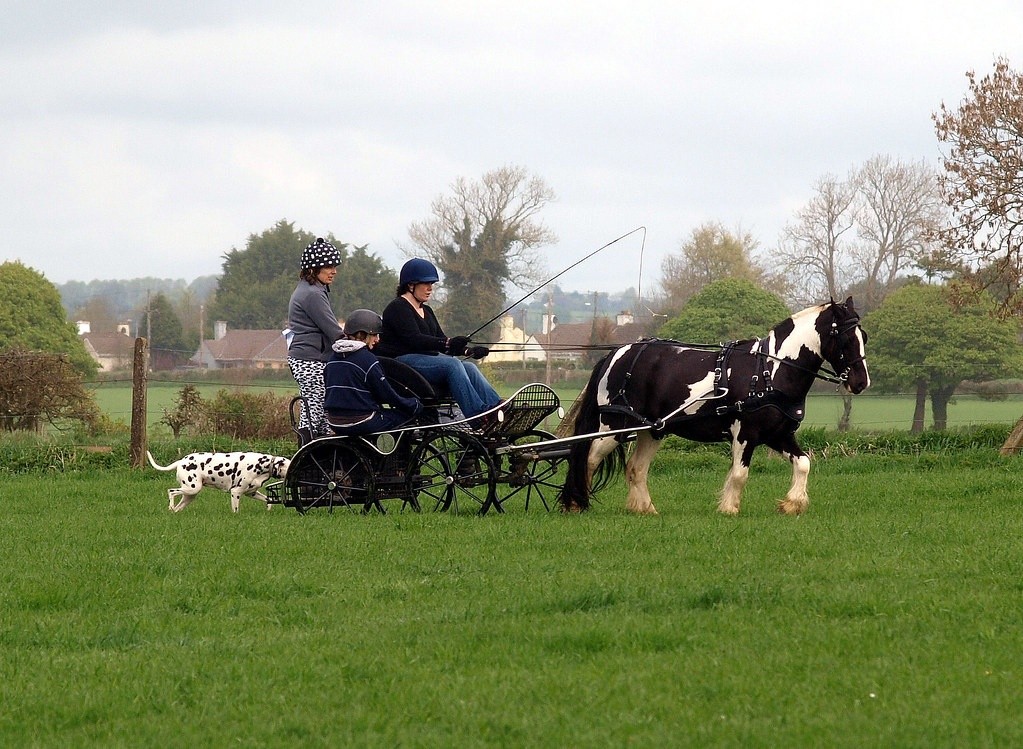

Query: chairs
[375,358,461,416]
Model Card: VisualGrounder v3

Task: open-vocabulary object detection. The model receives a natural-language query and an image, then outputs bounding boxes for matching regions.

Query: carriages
[265,295,871,517]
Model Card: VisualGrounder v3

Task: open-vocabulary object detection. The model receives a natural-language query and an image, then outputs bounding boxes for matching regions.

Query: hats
[400,257,439,283]
[343,308,384,334]
[300,238,342,269]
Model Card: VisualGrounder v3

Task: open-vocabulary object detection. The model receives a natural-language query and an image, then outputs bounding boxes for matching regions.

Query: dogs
[146,451,291,513]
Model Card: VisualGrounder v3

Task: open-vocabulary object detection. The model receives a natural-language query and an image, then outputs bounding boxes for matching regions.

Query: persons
[377,260,510,431]
[324,309,423,439]
[286,237,348,449]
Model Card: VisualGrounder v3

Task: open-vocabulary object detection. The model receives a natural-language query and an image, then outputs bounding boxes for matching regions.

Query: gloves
[466,345,489,359]
[448,335,472,351]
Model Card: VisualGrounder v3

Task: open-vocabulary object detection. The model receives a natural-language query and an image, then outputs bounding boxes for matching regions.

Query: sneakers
[465,398,509,429]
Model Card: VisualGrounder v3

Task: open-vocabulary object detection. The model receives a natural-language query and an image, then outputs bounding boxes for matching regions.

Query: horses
[551,296,871,517]
